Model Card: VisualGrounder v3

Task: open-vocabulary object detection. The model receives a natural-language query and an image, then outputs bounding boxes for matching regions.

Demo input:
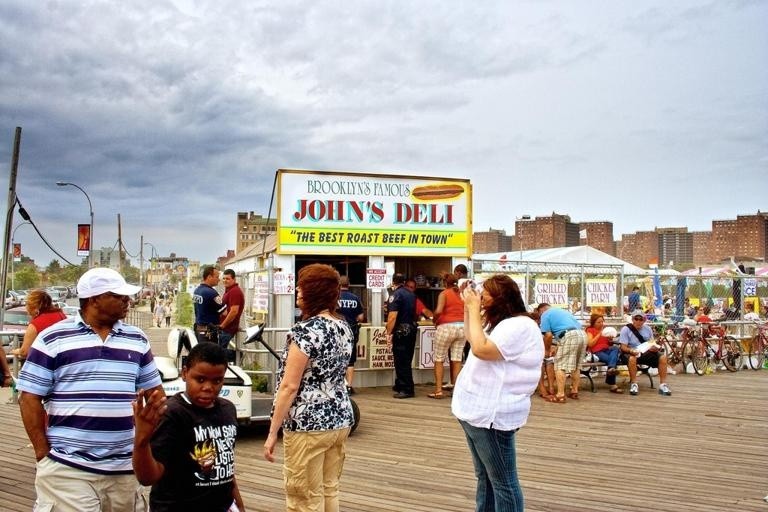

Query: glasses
[635,317,643,321]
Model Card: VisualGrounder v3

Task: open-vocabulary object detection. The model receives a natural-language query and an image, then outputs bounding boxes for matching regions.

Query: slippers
[610,387,623,394]
[608,368,619,376]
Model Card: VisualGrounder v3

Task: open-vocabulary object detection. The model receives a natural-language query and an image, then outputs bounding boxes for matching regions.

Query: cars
[565,295,673,310]
[0,283,157,360]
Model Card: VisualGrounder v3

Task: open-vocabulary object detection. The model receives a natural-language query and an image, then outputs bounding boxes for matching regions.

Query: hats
[632,311,645,317]
[76,267,142,300]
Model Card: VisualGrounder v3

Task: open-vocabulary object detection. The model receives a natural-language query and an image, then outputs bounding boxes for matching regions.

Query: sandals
[567,391,578,399]
[546,394,567,403]
[428,391,443,399]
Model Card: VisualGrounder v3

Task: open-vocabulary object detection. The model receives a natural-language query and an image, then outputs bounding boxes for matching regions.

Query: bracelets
[3,375,12,379]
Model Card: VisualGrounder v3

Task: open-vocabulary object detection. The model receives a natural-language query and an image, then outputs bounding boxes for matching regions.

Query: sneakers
[393,391,414,398]
[658,383,671,393]
[630,383,639,394]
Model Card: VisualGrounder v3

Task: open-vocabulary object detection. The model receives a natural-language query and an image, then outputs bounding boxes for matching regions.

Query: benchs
[581,341,656,393]
[148,327,253,387]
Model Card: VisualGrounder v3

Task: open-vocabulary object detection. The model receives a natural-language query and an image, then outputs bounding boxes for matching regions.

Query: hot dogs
[412,185,464,199]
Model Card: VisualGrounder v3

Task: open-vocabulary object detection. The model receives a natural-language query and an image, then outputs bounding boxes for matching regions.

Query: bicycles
[639,316,743,376]
[748,318,768,371]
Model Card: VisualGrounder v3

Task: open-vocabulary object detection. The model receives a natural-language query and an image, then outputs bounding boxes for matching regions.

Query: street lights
[144,243,153,300]
[620,242,635,261]
[516,215,531,265]
[56,181,93,270]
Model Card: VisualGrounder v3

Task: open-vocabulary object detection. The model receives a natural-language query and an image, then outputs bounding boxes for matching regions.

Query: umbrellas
[495,244,647,317]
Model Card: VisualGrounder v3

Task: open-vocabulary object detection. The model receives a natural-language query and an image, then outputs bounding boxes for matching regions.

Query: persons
[448,275,545,511]
[12,289,67,360]
[16,268,166,512]
[147,284,181,328]
[129,341,246,510]
[385,264,480,400]
[0,339,13,388]
[530,286,766,402]
[193,266,245,363]
[263,264,354,511]
[333,274,364,395]
